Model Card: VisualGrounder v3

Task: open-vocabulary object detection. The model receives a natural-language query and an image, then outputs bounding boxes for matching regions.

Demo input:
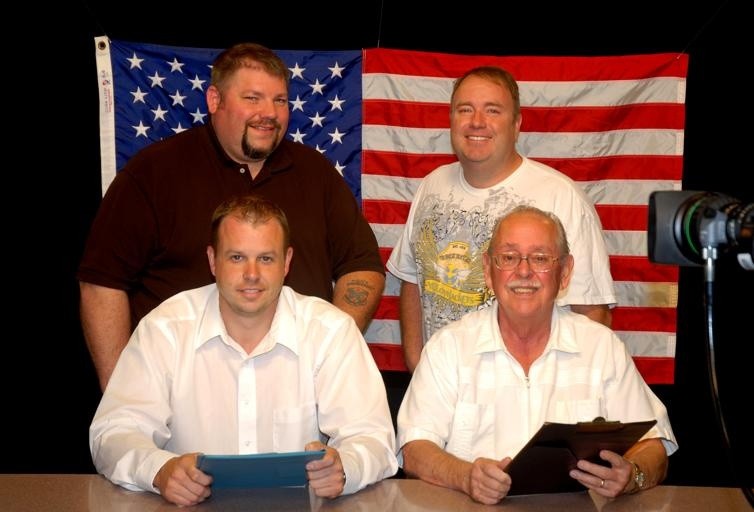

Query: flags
[94,34,691,385]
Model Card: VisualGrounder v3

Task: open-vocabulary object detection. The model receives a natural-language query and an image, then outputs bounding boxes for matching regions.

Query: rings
[600,478,606,489]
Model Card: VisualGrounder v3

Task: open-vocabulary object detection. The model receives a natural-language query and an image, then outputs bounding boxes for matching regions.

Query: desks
[1,472,754,512]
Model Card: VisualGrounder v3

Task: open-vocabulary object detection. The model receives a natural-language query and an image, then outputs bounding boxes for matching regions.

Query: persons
[384,64,619,377]
[394,204,680,507]
[87,194,400,510]
[75,41,386,394]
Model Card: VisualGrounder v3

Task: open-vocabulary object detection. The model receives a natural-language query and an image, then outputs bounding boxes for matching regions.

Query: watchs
[628,461,646,497]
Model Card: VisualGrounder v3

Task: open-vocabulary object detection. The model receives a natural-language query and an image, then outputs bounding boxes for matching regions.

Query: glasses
[491,250,563,273]
